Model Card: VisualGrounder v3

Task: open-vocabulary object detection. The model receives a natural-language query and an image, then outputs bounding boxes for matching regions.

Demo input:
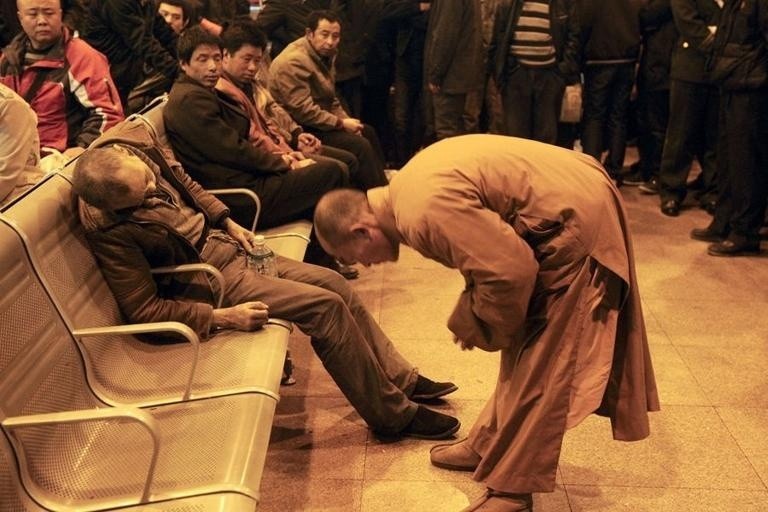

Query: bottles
[246,235,277,279]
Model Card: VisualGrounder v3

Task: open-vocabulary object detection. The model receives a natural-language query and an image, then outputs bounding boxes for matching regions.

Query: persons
[1,0,768,256]
[312,133,661,511]
[72,119,461,440]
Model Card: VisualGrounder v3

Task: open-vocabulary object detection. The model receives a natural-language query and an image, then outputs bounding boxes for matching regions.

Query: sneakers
[623,169,647,185]
[400,406,462,439]
[412,374,459,399]
[460,487,534,511]
[638,175,659,195]
[429,437,482,472]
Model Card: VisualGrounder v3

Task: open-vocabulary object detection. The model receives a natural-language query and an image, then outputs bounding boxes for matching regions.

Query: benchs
[0,96,313,512]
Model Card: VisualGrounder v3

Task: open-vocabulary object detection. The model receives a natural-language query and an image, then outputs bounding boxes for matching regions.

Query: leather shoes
[691,228,726,242]
[661,200,679,217]
[703,192,719,211]
[328,258,360,278]
[707,239,760,256]
